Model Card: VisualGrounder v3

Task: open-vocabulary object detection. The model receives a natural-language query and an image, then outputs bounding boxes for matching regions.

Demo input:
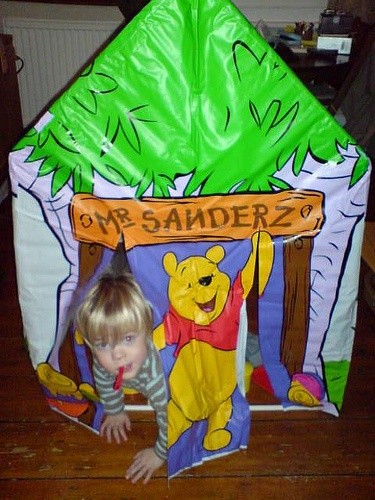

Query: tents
[5,0,371,480]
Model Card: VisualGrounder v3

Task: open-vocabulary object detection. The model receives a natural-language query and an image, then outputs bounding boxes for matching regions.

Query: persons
[69,270,172,487]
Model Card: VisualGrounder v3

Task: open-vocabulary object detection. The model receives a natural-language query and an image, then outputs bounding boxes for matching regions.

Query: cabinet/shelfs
[277,32,372,120]
